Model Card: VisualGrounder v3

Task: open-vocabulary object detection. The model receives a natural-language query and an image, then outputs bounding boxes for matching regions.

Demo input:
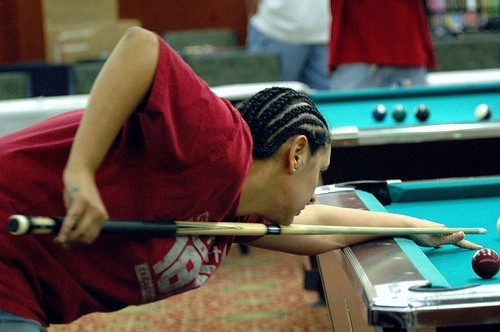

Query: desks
[308,80,500,185]
[304,176,500,332]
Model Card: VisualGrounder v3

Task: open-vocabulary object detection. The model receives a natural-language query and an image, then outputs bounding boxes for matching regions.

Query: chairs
[0,27,500,140]
[0,30,283,100]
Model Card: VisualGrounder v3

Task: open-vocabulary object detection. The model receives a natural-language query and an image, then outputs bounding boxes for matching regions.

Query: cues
[6,215,488,236]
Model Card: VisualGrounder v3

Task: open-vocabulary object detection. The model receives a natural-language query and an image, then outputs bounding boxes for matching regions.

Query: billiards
[415,105,429,121]
[475,104,491,120]
[372,104,386,120]
[472,248,500,279]
[393,105,406,121]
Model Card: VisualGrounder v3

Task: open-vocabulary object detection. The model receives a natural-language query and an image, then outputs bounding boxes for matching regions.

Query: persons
[0,26,483,332]
[329,0,437,90]
[246,0,333,91]
